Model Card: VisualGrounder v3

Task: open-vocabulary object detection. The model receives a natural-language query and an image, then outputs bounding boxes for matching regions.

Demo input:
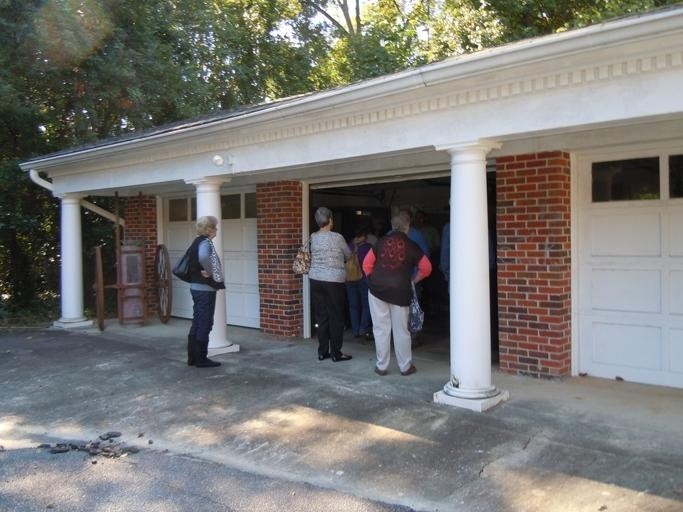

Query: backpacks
[343,242,366,283]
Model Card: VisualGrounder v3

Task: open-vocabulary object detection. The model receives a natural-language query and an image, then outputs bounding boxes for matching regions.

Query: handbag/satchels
[406,280,424,333]
[291,238,311,275]
[172,248,192,283]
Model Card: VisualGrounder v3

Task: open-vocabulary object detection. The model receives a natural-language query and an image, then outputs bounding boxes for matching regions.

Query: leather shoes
[318,354,330,360]
[331,354,351,362]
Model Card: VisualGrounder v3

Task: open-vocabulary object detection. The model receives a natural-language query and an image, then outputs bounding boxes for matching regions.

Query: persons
[344,198,449,348]
[307,206,354,362]
[361,211,433,376]
[185,215,226,368]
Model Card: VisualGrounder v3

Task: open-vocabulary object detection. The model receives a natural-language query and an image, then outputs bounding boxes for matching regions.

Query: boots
[187,336,196,365]
[195,343,220,367]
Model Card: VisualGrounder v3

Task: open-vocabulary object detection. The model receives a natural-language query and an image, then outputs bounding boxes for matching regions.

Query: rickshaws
[89,189,174,333]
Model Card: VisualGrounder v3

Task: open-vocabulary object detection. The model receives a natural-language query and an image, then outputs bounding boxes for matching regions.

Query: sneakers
[374,368,385,375]
[360,332,373,340]
[401,366,415,375]
